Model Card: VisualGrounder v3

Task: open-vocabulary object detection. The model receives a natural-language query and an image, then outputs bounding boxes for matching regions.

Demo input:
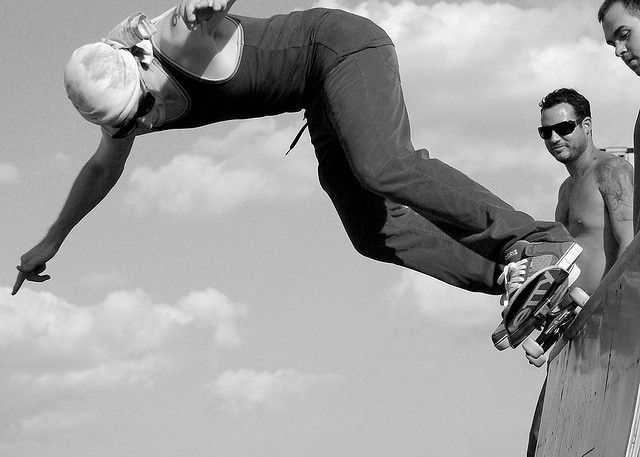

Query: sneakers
[490,260,583,352]
[496,235,585,349]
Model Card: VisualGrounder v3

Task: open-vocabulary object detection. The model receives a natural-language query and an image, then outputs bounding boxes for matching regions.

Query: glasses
[107,81,156,140]
[536,117,583,139]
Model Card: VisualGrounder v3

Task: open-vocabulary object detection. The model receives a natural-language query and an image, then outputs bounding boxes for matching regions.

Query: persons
[598,1,639,233]
[11,0,583,352]
[538,88,634,296]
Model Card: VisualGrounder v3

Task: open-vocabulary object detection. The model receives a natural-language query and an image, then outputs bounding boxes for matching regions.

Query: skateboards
[501,263,590,368]
